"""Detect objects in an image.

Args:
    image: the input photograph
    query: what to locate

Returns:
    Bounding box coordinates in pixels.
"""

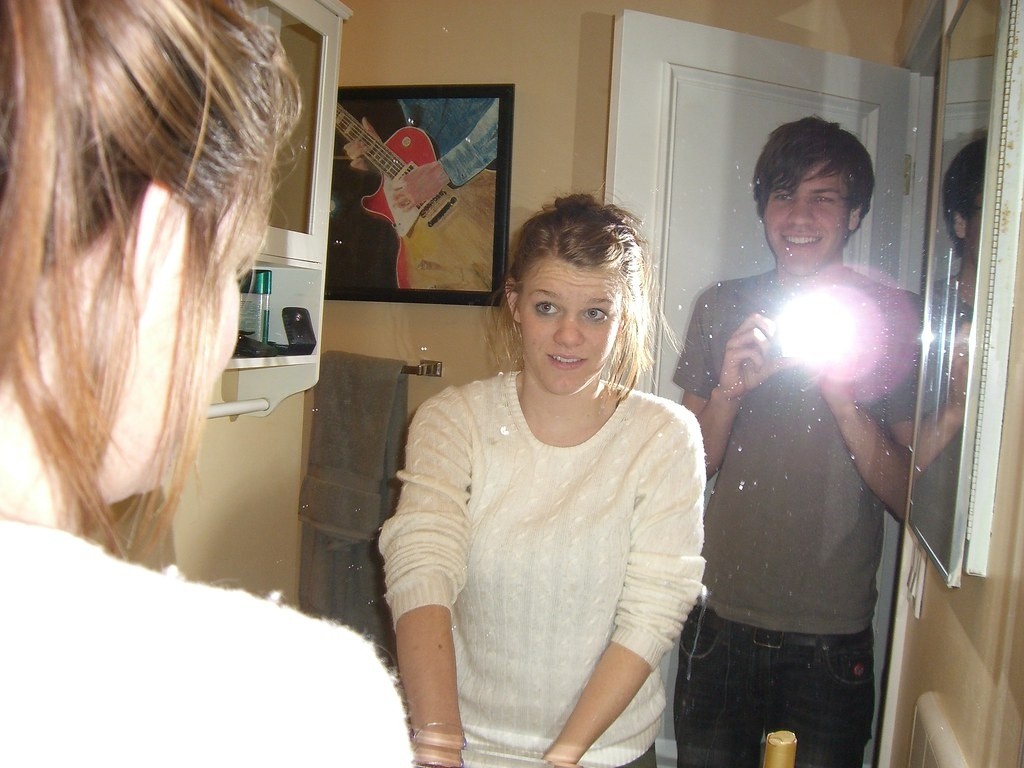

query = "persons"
[909,137,992,571]
[0,0,417,768]
[377,195,706,768]
[675,116,924,768]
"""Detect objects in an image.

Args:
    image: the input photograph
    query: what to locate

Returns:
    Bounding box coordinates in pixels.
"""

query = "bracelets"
[409,723,465,768]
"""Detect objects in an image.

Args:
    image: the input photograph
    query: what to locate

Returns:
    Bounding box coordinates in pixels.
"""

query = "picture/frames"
[322,82,517,306]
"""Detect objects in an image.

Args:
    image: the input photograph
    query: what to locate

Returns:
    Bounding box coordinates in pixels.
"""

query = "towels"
[297,350,410,662]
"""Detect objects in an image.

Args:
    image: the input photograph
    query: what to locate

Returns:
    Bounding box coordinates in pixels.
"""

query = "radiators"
[908,690,969,768]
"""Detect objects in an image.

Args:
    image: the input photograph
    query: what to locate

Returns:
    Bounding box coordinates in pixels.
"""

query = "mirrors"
[905,0,1024,593]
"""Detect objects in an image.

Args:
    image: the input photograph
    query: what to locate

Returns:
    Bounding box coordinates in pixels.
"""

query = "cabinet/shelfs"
[206,1,356,423]
